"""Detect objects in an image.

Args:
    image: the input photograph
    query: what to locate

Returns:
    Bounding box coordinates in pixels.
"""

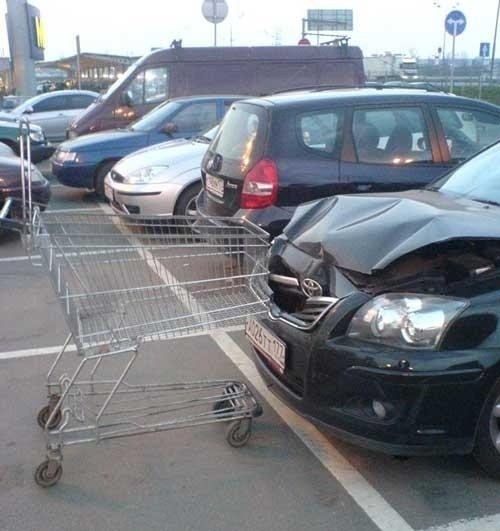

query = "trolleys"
[0,118,275,489]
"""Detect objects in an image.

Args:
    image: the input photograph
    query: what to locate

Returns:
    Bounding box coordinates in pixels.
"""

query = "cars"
[245,139,500,481]
[1,89,254,235]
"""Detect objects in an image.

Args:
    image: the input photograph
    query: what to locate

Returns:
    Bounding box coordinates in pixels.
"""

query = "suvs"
[194,82,500,261]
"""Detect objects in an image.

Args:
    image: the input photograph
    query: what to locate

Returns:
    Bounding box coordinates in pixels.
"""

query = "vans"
[67,37,366,139]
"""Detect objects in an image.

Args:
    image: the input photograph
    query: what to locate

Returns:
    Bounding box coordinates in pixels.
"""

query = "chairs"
[352,121,414,163]
